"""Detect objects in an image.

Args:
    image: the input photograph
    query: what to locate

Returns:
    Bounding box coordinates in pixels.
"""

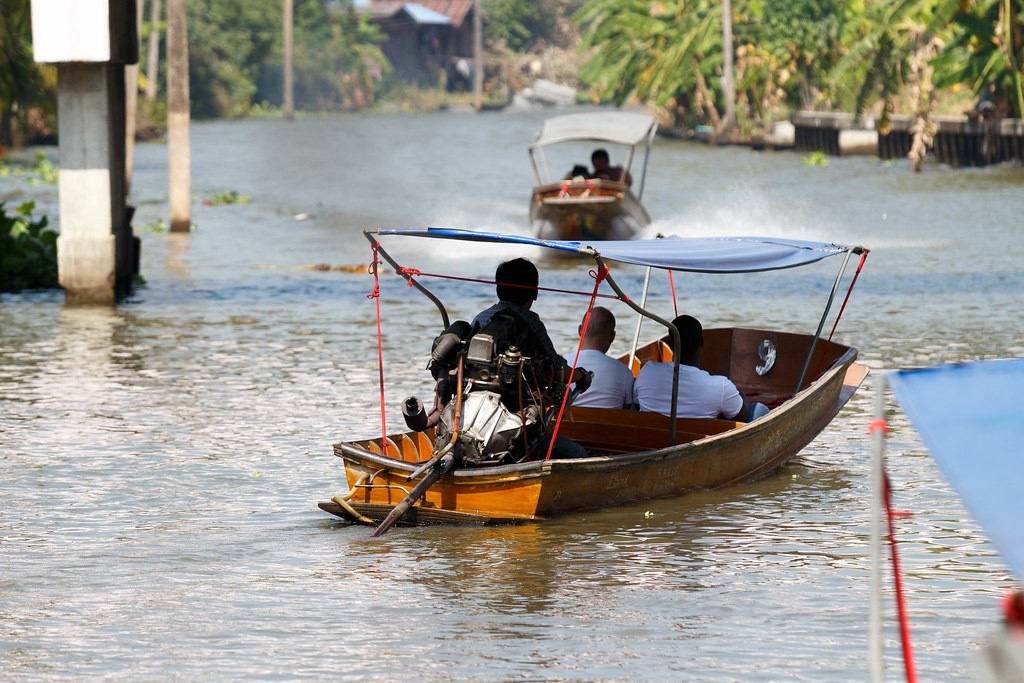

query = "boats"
[313,223,882,541]
[524,108,660,260]
[869,354,1024,683]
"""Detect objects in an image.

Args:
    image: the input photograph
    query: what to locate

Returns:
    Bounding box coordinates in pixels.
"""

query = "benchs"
[553,403,750,455]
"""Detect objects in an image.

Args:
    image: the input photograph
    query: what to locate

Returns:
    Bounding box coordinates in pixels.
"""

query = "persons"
[634,315,769,425]
[472,256,592,459]
[560,305,634,411]
[562,149,632,190]
